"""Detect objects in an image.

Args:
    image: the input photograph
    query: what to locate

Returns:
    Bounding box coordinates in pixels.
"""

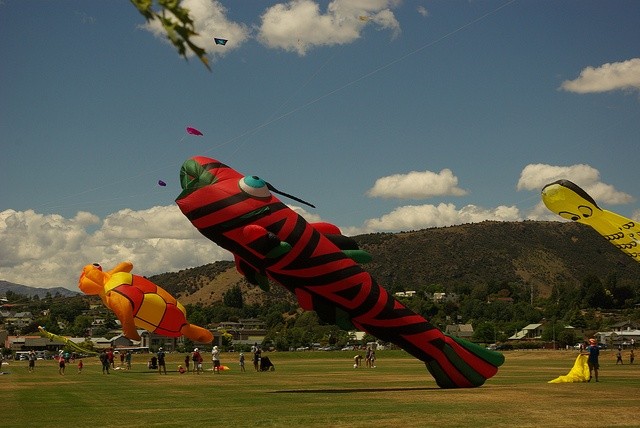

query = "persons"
[185,356,190,373]
[629,349,634,364]
[28,349,36,373]
[177,365,184,373]
[58,354,65,375]
[616,348,623,365]
[77,359,83,374]
[364,346,371,368]
[580,340,589,352]
[108,348,117,368]
[71,354,75,364]
[120,352,125,366]
[106,350,110,363]
[65,351,70,363]
[100,349,110,375]
[59,349,65,360]
[354,354,363,367]
[211,346,220,375]
[239,353,245,372]
[156,346,167,375]
[197,356,204,374]
[191,348,201,375]
[368,351,377,369]
[580,338,599,383]
[253,342,261,372]
[0,348,4,369]
[125,351,131,370]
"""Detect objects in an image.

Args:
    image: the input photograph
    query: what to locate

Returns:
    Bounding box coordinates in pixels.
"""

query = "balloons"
[37,326,99,355]
[78,261,214,345]
[174,156,505,389]
[541,179,640,262]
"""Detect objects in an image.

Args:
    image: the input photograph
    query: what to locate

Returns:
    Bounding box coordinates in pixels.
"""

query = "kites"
[359,15,371,22]
[187,127,203,136]
[159,180,166,186]
[130,0,212,72]
[214,38,228,46]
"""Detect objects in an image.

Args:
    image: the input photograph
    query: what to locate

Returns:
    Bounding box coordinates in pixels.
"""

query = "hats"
[194,348,198,351]
[213,346,218,350]
[589,339,596,344]
